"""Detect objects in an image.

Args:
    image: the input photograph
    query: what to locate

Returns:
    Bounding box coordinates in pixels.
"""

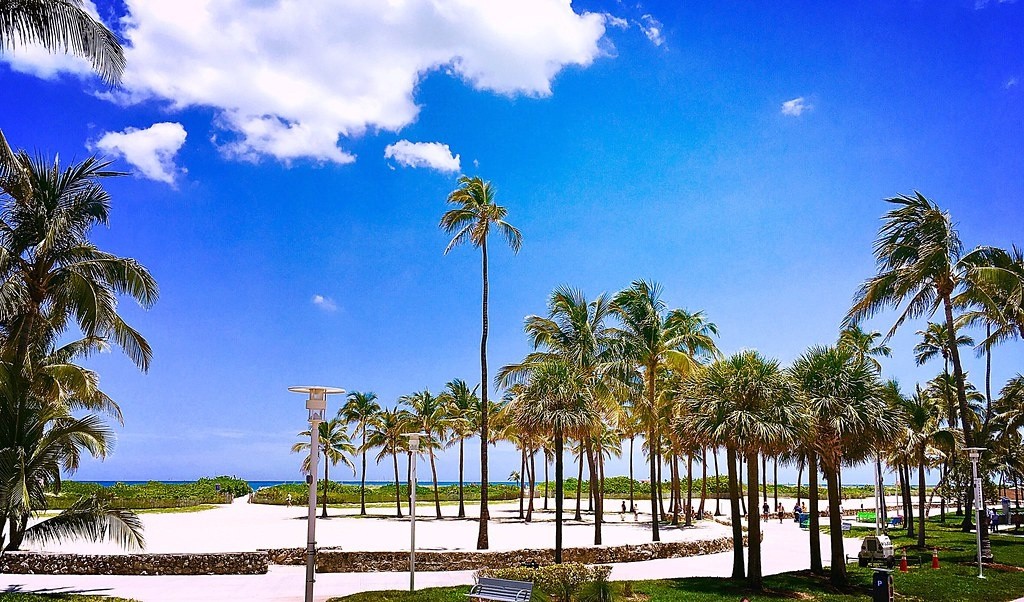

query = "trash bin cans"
[872,567,896,602]
[800,514,808,528]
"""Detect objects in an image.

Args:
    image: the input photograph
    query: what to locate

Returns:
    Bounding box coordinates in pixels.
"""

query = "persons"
[986,508,999,534]
[621,501,695,521]
[287,493,293,507]
[248,487,253,504]
[763,502,807,524]
[231,492,235,504]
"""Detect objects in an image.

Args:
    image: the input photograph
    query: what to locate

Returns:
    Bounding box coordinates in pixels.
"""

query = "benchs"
[463,577,533,602]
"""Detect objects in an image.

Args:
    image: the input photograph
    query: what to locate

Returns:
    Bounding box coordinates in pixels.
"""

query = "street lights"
[287,385,346,602]
[399,433,429,590]
[963,447,987,580]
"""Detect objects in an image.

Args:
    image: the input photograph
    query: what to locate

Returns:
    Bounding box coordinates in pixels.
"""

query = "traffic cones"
[930,546,941,569]
[899,547,909,573]
